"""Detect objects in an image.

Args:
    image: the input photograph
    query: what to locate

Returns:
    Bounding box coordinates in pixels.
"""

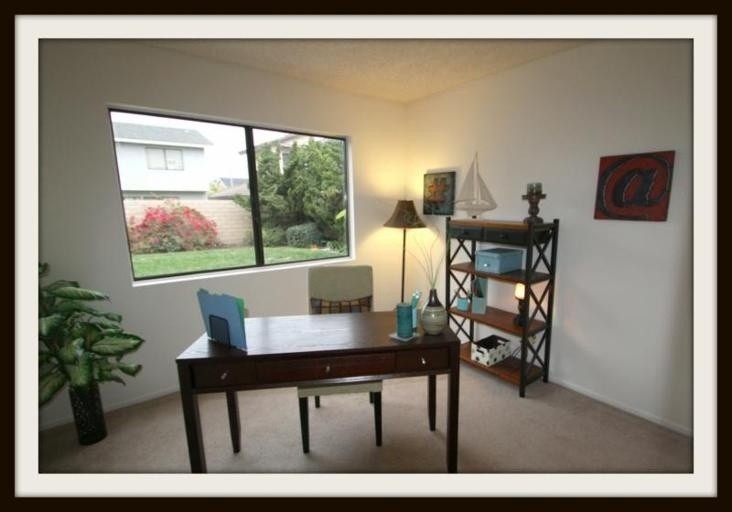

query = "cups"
[412,305,417,332]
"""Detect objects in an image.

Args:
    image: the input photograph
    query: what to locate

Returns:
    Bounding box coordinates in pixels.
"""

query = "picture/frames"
[423,172,455,215]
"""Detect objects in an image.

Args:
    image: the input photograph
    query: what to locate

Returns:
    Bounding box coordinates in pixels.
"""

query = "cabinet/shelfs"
[446,215,559,397]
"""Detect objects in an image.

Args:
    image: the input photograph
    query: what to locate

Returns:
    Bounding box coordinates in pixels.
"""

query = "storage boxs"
[475,248,523,274]
[471,334,512,367]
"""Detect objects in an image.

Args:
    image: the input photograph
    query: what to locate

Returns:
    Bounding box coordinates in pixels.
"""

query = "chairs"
[297,265,382,454]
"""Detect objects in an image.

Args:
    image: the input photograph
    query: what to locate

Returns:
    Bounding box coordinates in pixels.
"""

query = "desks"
[175,309,460,473]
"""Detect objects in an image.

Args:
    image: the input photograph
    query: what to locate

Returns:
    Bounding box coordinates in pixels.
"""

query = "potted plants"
[38,262,145,445]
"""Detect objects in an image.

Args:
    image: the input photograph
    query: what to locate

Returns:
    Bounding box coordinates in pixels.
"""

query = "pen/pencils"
[411,291,422,308]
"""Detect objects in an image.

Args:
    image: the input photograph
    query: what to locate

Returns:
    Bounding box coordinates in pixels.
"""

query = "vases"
[421,289,447,336]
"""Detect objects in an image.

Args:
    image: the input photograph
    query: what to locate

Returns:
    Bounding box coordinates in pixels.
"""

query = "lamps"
[514,282,530,327]
[384,200,427,303]
